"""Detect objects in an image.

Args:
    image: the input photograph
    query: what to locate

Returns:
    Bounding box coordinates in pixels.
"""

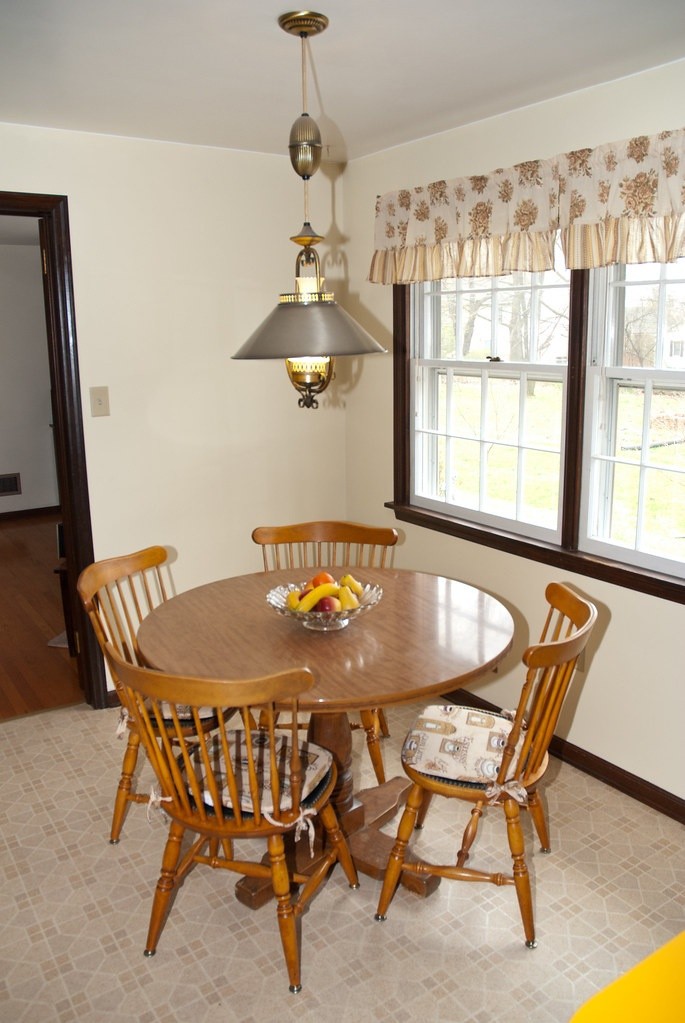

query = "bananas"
[286,583,341,613]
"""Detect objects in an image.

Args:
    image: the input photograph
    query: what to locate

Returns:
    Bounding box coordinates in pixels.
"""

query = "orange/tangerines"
[312,572,335,587]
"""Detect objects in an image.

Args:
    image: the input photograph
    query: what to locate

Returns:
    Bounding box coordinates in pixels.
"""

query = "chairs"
[104,642,363,993]
[77,544,239,844]
[252,521,402,784]
[374,582,599,948]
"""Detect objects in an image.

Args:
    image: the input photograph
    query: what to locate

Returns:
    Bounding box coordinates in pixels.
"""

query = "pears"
[338,574,363,611]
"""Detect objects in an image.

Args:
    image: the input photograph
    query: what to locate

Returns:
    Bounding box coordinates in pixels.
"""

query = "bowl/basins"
[265,582,385,631]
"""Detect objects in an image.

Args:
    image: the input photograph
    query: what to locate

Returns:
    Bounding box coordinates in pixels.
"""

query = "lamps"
[234,12,386,412]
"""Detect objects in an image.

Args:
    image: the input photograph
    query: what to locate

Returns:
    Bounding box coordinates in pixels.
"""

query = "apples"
[312,596,341,613]
[299,589,313,601]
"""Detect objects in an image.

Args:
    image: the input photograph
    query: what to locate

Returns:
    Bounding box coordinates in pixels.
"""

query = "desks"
[137,567,529,912]
